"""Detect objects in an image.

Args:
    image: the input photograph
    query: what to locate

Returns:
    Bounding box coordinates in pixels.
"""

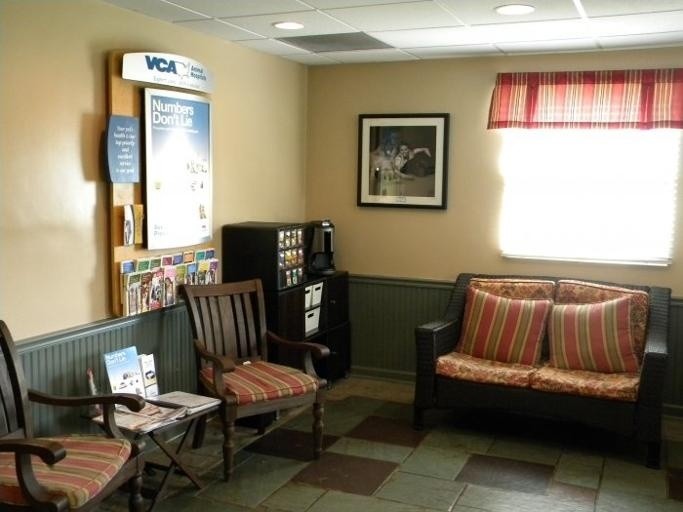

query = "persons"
[393,143,431,180]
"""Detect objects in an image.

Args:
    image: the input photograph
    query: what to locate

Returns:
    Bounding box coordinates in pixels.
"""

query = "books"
[91,345,222,435]
[119,247,218,315]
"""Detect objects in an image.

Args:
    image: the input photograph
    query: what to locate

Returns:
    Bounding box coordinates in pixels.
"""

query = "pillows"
[450,282,555,369]
[538,290,644,378]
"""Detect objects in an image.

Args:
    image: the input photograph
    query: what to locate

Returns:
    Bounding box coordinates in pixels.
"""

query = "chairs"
[0,318,153,512]
[177,275,331,484]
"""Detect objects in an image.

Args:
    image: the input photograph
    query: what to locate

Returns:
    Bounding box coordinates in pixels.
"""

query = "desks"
[75,385,223,512]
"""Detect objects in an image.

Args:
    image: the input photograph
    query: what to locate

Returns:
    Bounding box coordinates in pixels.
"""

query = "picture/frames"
[354,108,453,212]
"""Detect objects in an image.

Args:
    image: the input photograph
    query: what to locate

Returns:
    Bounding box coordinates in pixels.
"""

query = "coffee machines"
[307,219,335,276]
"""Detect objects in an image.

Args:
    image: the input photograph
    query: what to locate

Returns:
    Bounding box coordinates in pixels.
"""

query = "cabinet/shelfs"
[216,266,353,400]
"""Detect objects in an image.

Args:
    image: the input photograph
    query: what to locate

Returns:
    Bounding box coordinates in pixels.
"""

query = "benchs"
[407,267,675,470]
